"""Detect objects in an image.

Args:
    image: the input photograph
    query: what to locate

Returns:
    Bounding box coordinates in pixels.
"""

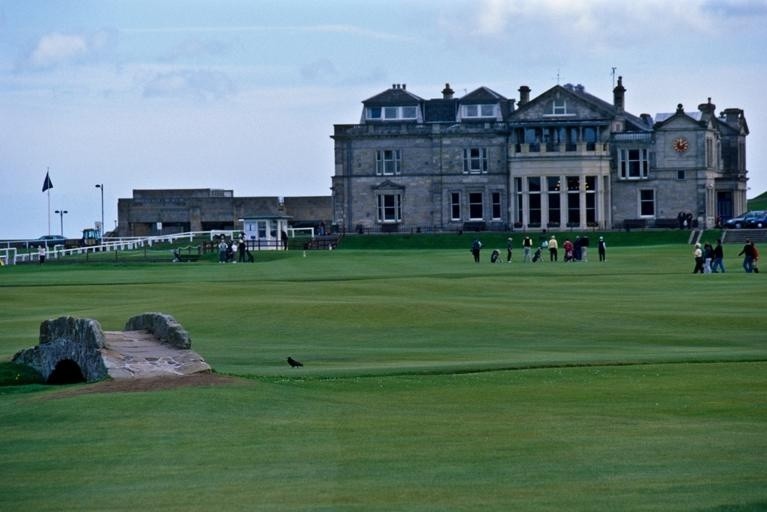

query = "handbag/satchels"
[752,260,758,269]
[491,249,500,263]
[532,247,542,263]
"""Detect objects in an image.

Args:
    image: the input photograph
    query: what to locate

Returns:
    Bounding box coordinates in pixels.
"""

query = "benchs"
[623,219,646,232]
[380,223,399,232]
[655,218,680,231]
[463,222,485,231]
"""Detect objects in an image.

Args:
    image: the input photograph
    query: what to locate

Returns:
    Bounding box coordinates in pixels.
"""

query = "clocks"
[672,136,689,153]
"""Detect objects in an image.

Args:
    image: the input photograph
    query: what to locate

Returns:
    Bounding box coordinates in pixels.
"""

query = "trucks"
[64,228,101,254]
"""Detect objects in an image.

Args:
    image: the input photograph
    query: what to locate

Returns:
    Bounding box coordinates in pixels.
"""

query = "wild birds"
[285,356,303,368]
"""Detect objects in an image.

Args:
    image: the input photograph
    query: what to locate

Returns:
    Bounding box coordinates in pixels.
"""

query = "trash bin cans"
[356,225,362,233]
[330,223,339,232]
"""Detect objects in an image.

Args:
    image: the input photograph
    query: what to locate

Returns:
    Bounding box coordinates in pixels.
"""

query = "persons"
[490,249,502,264]
[539,228,549,249]
[597,236,607,263]
[522,235,532,263]
[748,240,758,275]
[685,210,693,230]
[736,237,754,272]
[547,234,558,262]
[216,237,245,264]
[36,243,46,268]
[282,232,288,252]
[506,236,514,264]
[580,234,589,262]
[572,235,581,262]
[676,209,686,230]
[531,246,543,263]
[561,238,572,262]
[470,238,481,264]
[690,238,725,274]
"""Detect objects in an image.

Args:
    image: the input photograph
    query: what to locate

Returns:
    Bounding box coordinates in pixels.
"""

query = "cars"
[22,235,67,249]
[724,210,767,229]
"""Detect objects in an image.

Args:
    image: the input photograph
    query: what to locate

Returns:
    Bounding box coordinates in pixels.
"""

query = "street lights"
[94,183,104,247]
[54,210,68,235]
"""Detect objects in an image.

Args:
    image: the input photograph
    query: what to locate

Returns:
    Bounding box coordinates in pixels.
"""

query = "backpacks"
[564,240,572,251]
